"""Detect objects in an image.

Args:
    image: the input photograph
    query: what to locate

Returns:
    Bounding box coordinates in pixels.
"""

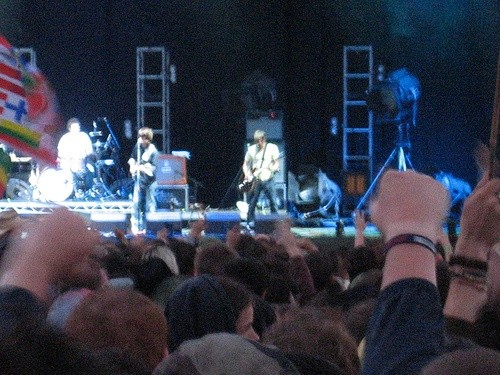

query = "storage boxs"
[150,185,189,212]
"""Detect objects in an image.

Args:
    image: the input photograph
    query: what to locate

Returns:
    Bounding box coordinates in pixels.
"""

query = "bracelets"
[447,253,490,292]
[383,235,438,257]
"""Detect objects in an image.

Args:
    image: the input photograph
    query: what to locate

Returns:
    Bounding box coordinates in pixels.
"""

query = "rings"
[201,226,205,231]
[356,215,359,217]
[86,225,102,238]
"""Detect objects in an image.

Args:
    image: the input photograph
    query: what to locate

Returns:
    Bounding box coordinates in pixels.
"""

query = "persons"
[55,117,102,199]
[0,141,500,375]
[127,125,157,237]
[241,129,281,231]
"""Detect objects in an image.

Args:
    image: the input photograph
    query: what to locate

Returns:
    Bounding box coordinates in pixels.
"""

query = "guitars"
[238,151,286,192]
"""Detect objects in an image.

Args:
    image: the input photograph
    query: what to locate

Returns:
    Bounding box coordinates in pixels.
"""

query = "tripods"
[78,135,116,201]
[352,123,415,219]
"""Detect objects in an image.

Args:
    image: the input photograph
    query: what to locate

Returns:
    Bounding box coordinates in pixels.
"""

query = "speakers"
[145,212,182,236]
[204,212,241,234]
[91,211,131,234]
[255,215,291,235]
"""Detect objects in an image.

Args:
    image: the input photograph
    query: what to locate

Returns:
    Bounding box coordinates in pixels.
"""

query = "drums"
[37,169,75,201]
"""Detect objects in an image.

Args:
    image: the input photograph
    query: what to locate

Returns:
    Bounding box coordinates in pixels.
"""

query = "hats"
[0,207,31,236]
[152,332,300,375]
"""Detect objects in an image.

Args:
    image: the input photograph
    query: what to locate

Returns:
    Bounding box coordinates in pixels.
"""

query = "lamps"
[354,67,422,210]
